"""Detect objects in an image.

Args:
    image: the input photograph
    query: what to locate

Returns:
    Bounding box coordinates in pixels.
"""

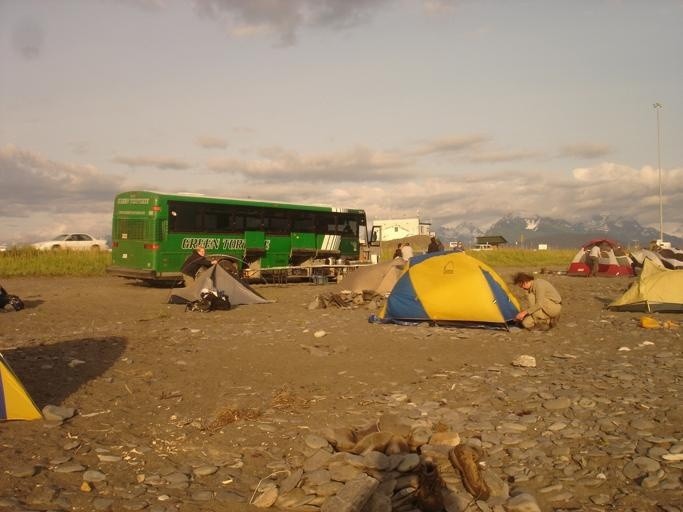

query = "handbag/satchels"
[187,291,231,312]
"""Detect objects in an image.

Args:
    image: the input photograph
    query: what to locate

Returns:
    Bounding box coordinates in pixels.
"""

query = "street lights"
[653,103,663,239]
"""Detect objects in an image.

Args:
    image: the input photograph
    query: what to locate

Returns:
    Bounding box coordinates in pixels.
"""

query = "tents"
[0,353,45,423]
[605,247,683,314]
[375,249,521,331]
[565,237,636,278]
[169,254,279,305]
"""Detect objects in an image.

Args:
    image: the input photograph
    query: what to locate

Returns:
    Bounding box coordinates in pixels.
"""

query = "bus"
[111,192,381,285]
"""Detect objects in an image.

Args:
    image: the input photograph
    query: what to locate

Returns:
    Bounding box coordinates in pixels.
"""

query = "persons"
[428,237,438,252]
[513,272,562,330]
[589,243,602,278]
[393,243,402,259]
[179,246,218,286]
[453,242,465,252]
[402,242,413,260]
[437,240,444,250]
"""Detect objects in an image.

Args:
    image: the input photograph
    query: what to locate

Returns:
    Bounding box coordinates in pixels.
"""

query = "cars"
[33,233,110,251]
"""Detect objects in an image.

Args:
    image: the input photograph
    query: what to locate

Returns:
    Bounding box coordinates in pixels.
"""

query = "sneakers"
[550,314,559,328]
[529,323,549,331]
